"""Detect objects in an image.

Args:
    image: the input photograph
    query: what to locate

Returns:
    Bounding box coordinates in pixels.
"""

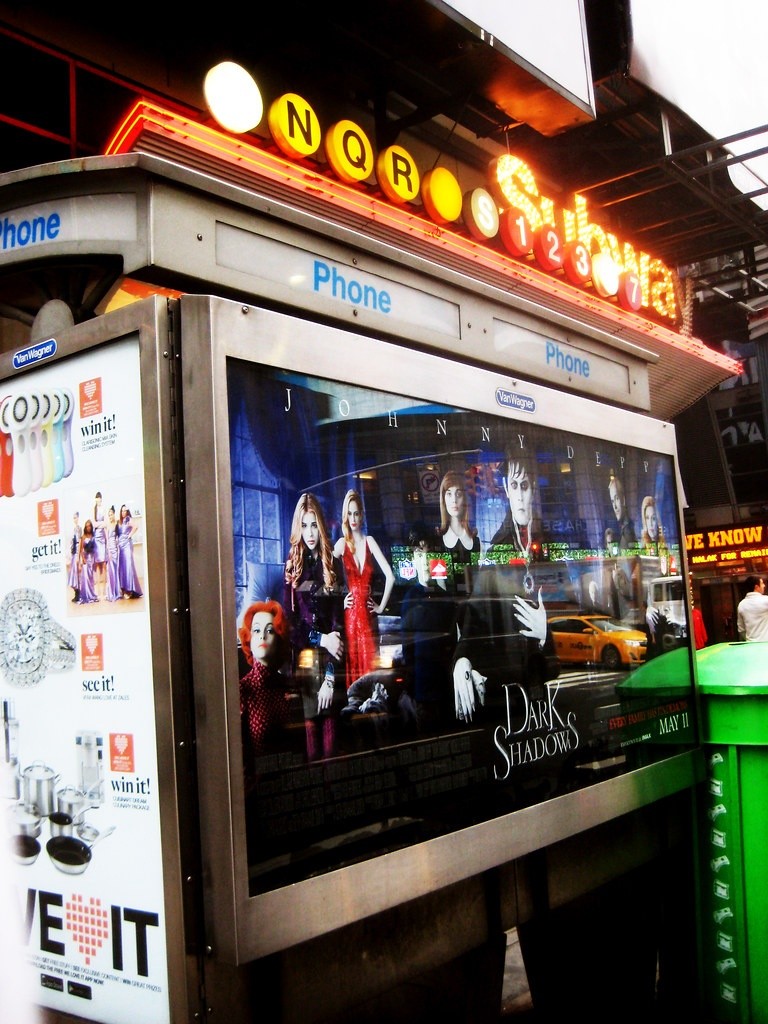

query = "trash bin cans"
[615,646,691,768]
[697,634,768,1023]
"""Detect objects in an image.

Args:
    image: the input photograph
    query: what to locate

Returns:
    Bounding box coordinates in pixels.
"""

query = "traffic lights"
[533,544,543,561]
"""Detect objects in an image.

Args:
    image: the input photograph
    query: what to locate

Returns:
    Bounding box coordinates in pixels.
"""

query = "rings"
[372,603,374,606]
[464,671,472,680]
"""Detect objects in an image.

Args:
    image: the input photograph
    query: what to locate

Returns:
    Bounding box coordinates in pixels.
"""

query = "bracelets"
[324,676,335,688]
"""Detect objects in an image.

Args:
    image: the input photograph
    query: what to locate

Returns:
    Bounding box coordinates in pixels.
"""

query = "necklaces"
[512,518,535,594]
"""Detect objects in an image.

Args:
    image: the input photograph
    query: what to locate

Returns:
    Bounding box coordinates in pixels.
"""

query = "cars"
[649,576,685,625]
[548,615,648,666]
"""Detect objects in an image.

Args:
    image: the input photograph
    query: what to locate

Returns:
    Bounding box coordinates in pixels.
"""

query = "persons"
[66,491,142,604]
[236,442,677,766]
[736,576,768,642]
